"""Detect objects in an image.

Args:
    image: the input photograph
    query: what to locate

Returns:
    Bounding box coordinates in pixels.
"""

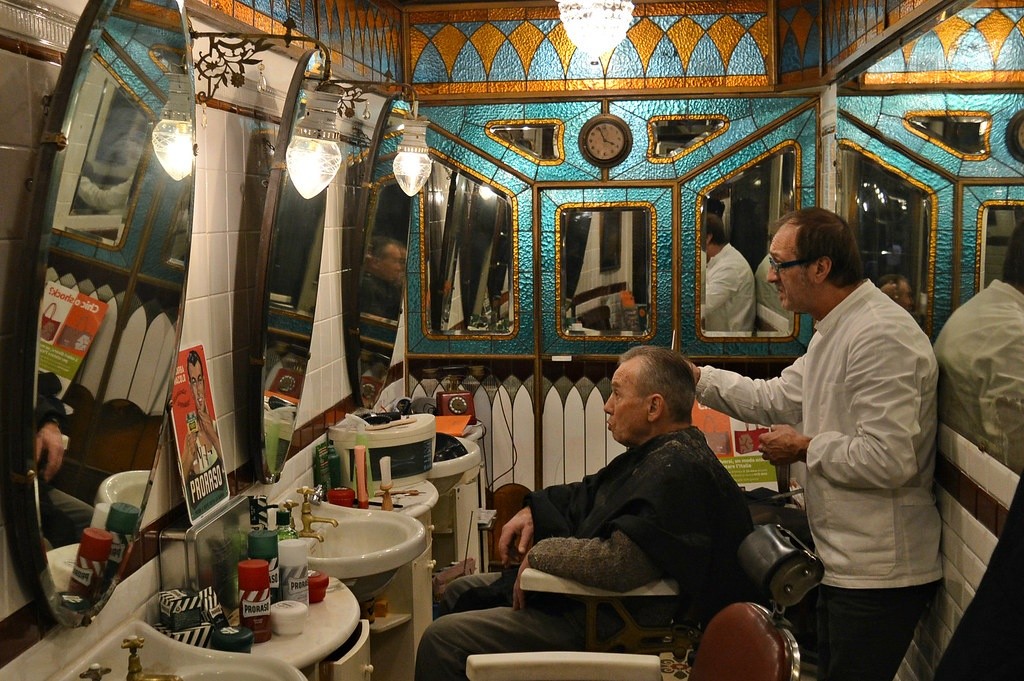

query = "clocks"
[1004,108,1024,164]
[579,113,633,170]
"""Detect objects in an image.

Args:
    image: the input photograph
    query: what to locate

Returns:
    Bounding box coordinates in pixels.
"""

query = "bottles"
[67,502,142,614]
[275,510,299,541]
[276,539,312,608]
[249,530,281,610]
[237,558,273,644]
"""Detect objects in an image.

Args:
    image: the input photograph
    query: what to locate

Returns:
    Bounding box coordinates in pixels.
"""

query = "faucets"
[79,663,112,681]
[122,636,182,681]
[297,485,338,542]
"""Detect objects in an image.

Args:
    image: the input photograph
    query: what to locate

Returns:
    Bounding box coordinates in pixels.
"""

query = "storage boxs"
[154,588,230,652]
[37,279,109,400]
[714,430,779,496]
[314,439,341,499]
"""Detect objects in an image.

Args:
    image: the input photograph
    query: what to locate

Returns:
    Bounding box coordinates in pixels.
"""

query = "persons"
[700,214,756,333]
[414,347,754,681]
[77,173,134,212]
[359,236,406,318]
[34,389,95,546]
[933,217,1024,476]
[875,275,911,312]
[755,252,789,317]
[682,207,944,681]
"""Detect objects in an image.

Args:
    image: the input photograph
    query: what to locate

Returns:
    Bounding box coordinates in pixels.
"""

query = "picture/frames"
[599,210,621,272]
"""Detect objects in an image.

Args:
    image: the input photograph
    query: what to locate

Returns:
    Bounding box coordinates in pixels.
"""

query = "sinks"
[307,507,427,579]
[433,437,481,476]
[183,642,309,681]
[95,469,150,506]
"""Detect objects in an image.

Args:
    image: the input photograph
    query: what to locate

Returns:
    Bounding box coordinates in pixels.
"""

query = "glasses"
[769,253,835,275]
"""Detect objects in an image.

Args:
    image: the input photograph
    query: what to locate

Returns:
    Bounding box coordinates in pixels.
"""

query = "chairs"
[467,495,827,681]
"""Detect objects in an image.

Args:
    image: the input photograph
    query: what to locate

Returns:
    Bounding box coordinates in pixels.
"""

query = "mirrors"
[836,137,938,342]
[51,50,159,250]
[5,0,657,627]
[484,119,565,166]
[160,183,194,274]
[647,0,1024,476]
[973,200,1024,294]
[356,172,410,329]
[904,110,992,161]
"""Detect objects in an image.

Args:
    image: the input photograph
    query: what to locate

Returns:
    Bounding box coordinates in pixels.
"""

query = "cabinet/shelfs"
[300,469,482,681]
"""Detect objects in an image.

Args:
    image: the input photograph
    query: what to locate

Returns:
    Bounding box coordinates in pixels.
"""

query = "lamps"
[190,19,344,201]
[330,79,432,197]
[149,41,194,181]
[555,0,636,65]
